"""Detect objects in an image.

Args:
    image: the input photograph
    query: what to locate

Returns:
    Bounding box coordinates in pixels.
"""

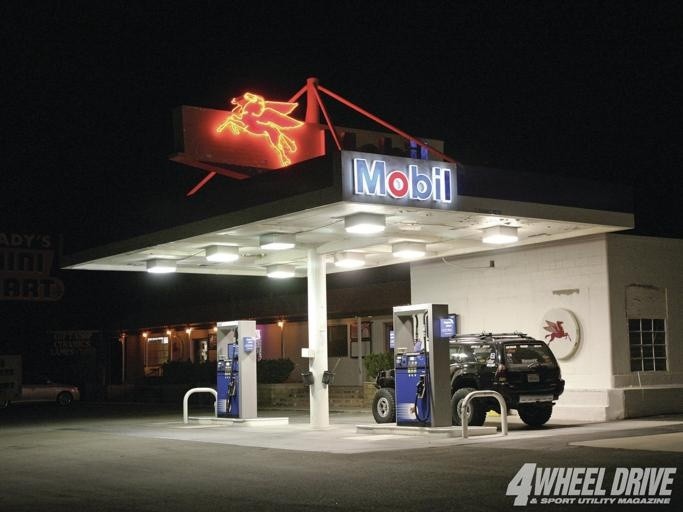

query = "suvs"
[372,333,565,426]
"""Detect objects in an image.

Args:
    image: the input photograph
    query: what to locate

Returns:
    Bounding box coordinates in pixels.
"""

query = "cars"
[0,375,81,409]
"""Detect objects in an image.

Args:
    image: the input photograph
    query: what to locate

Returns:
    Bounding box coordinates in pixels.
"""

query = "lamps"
[481,225,518,245]
[146,212,427,279]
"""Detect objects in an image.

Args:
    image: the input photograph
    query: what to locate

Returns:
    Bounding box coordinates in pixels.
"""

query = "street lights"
[119,332,126,384]
